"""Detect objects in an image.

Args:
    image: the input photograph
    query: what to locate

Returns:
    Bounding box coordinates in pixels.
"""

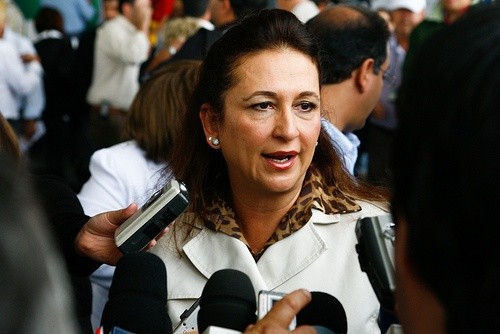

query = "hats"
[392,0,427,14]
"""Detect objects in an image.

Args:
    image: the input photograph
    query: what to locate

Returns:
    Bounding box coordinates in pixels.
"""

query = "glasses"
[373,63,398,87]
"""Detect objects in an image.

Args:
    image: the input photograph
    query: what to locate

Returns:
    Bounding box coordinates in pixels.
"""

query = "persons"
[27,8,75,133]
[370,0,425,149]
[98,10,410,334]
[88,0,151,147]
[0,1,500,333]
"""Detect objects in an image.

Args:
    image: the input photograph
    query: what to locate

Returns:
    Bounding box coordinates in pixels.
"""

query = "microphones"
[100,252,258,334]
[295,291,347,334]
